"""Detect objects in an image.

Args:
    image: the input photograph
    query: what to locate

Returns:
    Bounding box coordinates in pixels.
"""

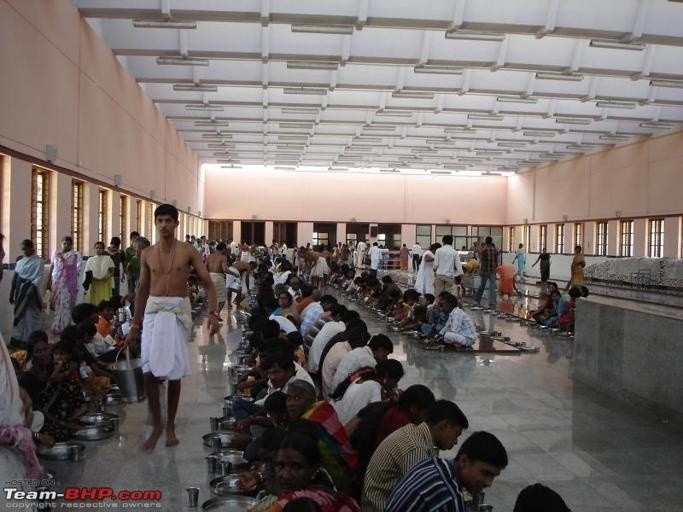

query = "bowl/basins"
[41,386,121,461]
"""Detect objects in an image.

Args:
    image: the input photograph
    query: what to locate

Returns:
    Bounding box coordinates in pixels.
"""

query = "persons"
[0,204,589,512]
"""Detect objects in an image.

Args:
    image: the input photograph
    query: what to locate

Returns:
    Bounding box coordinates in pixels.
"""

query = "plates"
[474,323,538,353]
[466,303,574,339]
[353,298,439,350]
[199,343,253,511]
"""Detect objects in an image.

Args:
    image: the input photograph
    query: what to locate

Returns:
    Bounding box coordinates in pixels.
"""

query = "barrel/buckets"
[478,330,495,352]
[106,343,146,404]
[229,276,242,293]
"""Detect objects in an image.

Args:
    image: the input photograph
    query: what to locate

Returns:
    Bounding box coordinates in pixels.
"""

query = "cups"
[184,484,199,508]
